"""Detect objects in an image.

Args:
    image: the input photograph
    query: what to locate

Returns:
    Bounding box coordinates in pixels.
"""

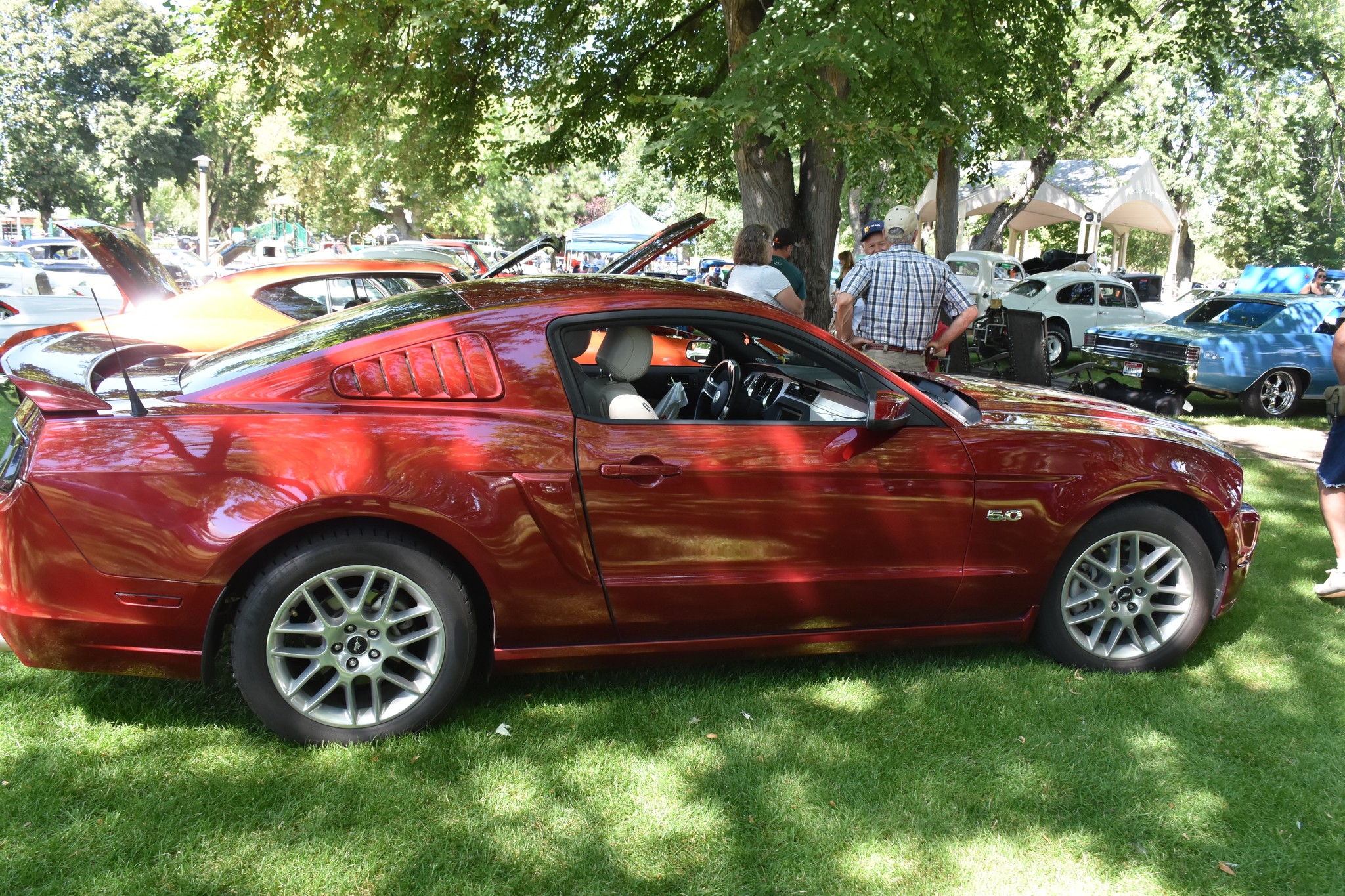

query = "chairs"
[1007,308,1098,395]
[582,323,659,420]
[561,327,592,388]
[940,308,1009,378]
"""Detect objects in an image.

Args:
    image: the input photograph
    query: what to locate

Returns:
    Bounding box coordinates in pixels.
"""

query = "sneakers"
[1313,569,1345,598]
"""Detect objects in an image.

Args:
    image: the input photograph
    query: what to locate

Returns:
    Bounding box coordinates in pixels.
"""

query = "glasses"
[1315,275,1327,278]
[768,241,775,247]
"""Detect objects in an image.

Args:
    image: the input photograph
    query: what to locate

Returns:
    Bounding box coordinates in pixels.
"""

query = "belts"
[865,344,926,356]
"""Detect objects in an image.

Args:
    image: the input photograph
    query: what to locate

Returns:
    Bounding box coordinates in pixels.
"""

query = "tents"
[563,201,697,281]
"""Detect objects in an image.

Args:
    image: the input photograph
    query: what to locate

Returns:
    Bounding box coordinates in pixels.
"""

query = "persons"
[60,251,71,260]
[927,320,949,372]
[201,253,227,281]
[188,241,197,253]
[829,250,865,337]
[860,221,889,304]
[1071,267,1102,302]
[725,223,803,315]
[1314,312,1345,598]
[769,228,808,319]
[835,206,979,372]
[1110,267,1125,294]
[1300,268,1327,295]
[556,252,690,281]
[705,267,722,286]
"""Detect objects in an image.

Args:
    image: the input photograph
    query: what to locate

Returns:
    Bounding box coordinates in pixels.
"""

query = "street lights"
[192,154,213,266]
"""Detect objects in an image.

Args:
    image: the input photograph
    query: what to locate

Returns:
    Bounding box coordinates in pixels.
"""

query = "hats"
[714,267,721,275]
[884,205,920,239]
[861,220,884,242]
[773,228,801,247]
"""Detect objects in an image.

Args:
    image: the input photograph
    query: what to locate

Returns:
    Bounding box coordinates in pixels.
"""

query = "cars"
[2,273,1259,748]
[972,270,1148,371]
[943,251,1032,330]
[1114,272,1240,323]
[1078,265,1345,415]
[0,212,806,420]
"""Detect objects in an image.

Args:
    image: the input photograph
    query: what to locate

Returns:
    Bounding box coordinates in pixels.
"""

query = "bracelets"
[841,330,854,341]
